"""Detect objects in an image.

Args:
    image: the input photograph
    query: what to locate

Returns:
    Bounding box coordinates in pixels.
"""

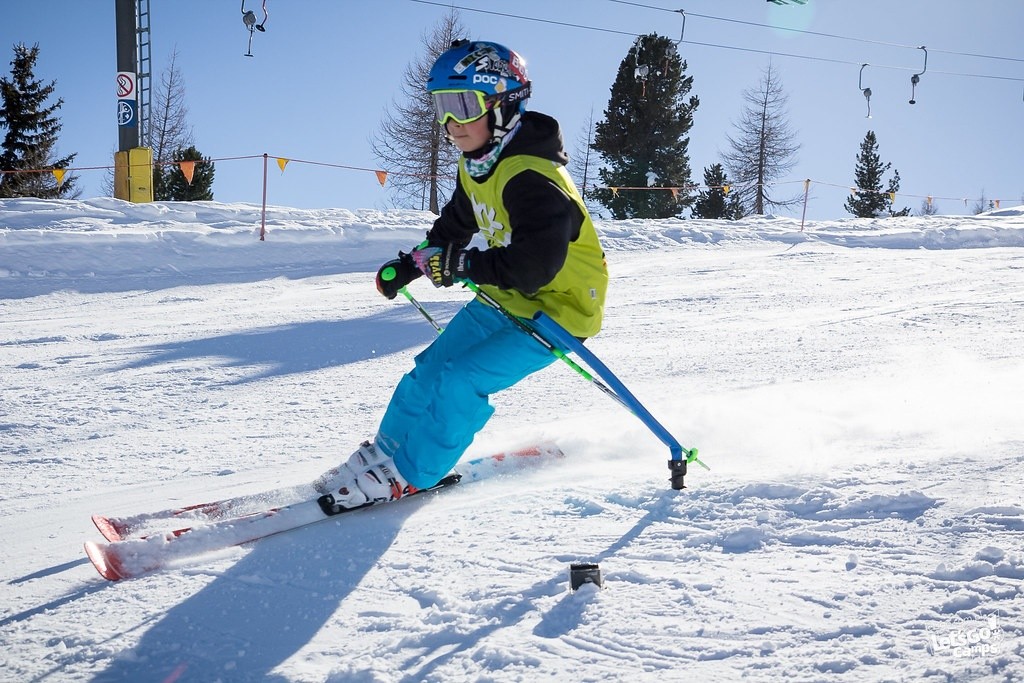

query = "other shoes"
[348,440,390,476]
[356,457,409,500]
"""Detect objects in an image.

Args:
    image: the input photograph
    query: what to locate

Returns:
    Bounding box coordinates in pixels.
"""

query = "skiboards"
[81,438,564,582]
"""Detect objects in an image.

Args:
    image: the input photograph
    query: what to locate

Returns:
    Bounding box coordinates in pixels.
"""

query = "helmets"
[426,39,534,147]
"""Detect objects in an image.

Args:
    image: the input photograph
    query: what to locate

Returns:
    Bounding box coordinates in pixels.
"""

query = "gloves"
[375,250,416,300]
[413,243,469,288]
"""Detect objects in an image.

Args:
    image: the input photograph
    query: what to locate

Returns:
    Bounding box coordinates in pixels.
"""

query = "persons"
[313,35,608,506]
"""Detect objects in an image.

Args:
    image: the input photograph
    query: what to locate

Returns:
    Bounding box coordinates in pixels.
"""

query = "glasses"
[431,89,494,126]
